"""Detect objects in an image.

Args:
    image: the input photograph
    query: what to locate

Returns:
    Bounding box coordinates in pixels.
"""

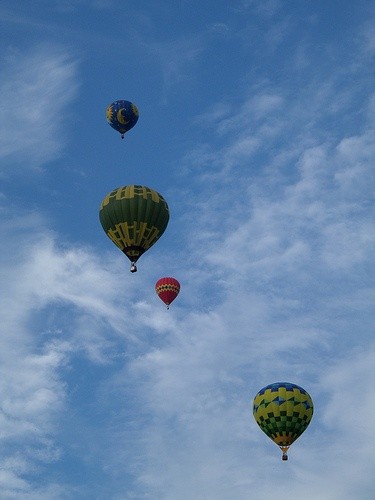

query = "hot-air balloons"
[252,382,315,461]
[106,100,140,139]
[97,184,169,273]
[154,277,181,310]
